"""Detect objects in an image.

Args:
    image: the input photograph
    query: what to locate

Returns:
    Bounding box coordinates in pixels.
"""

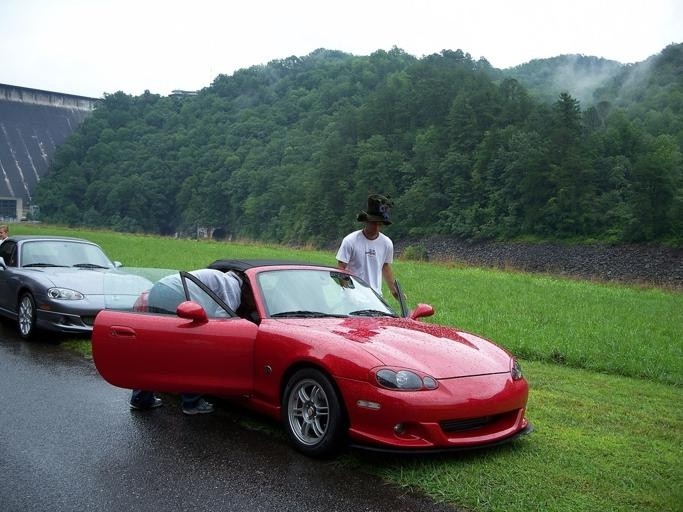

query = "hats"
[355,190,394,226]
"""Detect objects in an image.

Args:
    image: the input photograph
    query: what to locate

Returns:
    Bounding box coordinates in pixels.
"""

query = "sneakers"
[182,399,216,415]
[129,395,163,410]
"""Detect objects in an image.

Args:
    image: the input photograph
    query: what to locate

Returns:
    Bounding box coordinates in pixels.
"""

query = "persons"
[0,225,9,244]
[336,220,406,304]
[128,267,242,414]
